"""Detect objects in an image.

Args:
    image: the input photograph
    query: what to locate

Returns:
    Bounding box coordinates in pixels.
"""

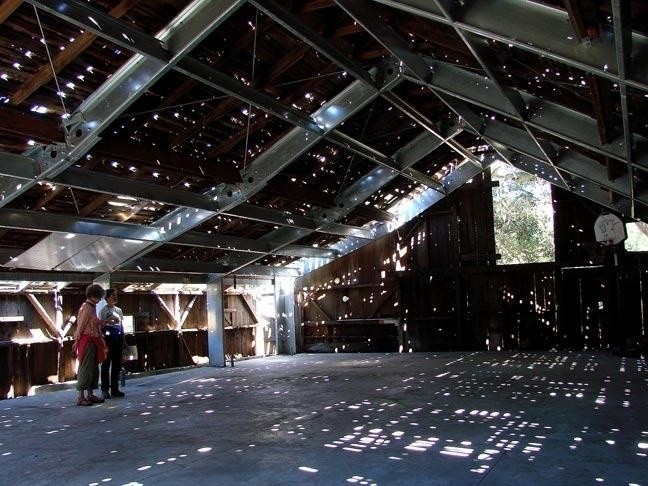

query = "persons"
[97,288,128,398]
[71,284,105,406]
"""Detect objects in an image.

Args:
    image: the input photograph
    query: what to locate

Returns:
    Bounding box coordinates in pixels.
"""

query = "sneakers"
[77,390,125,406]
